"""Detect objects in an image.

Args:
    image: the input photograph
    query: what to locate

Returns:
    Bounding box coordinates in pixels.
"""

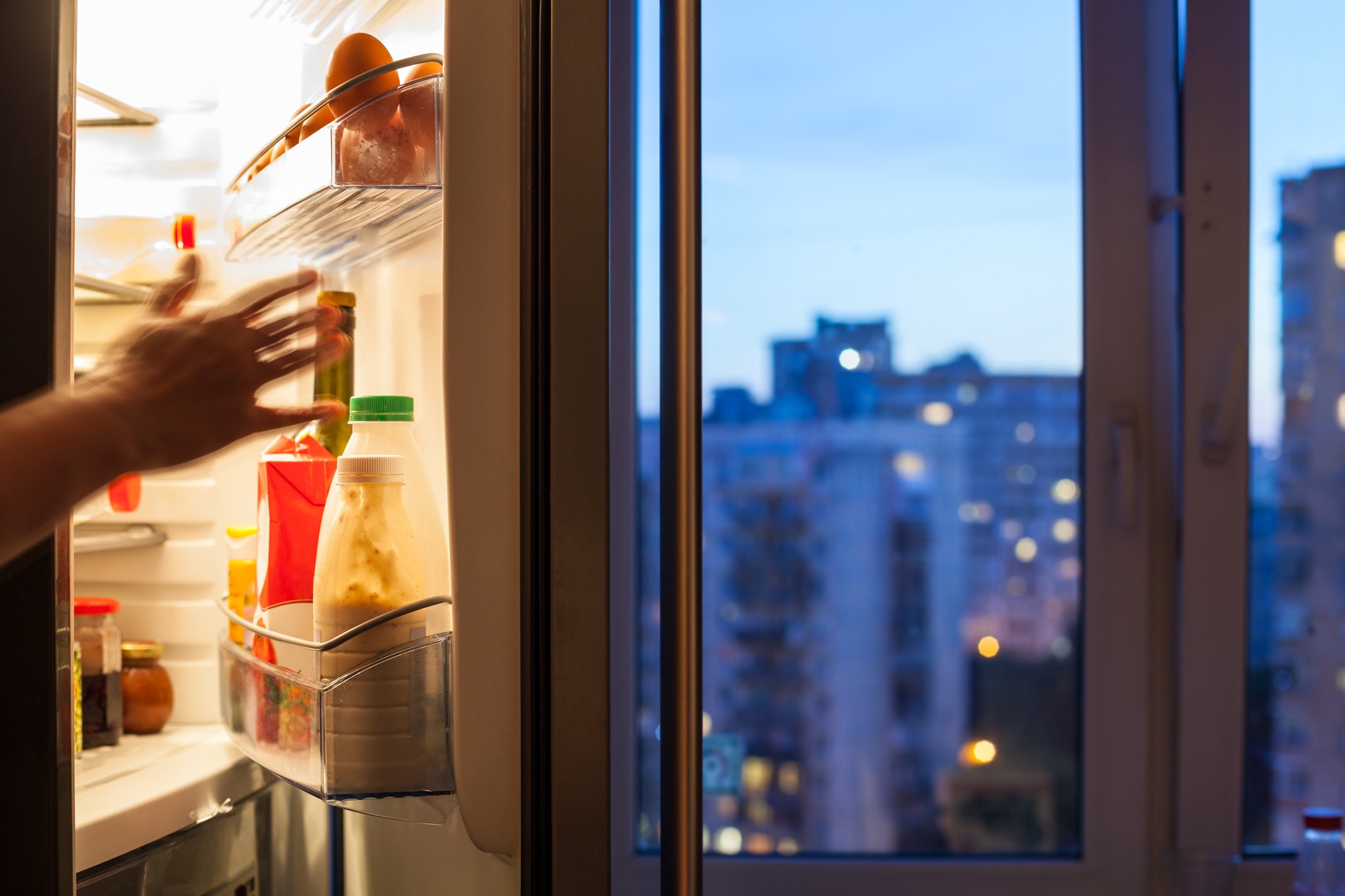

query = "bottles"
[313,292,354,457]
[315,455,430,800]
[317,395,453,697]
[73,596,124,749]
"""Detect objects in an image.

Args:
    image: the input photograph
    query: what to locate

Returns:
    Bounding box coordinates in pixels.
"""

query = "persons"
[0,253,352,562]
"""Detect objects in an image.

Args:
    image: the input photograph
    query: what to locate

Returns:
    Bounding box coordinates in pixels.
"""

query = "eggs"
[249,33,443,184]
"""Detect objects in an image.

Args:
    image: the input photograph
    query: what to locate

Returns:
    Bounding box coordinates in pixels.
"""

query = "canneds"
[121,639,174,736]
